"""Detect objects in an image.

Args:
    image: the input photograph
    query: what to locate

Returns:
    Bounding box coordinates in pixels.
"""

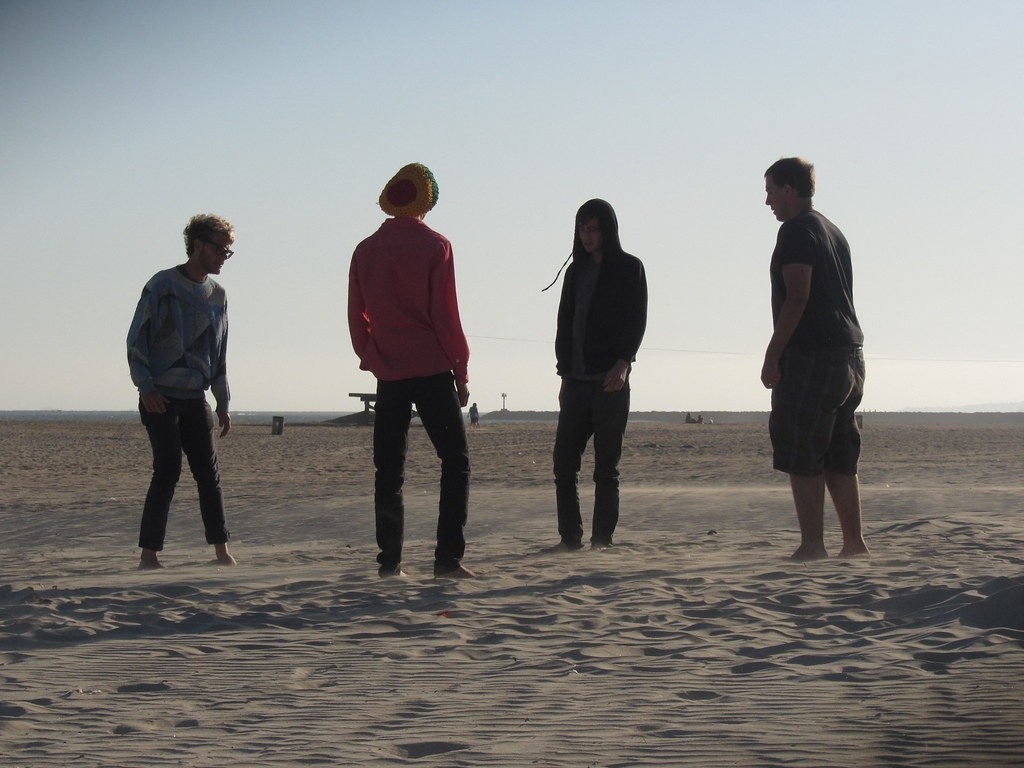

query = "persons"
[761,158,866,558]
[348,163,476,580]
[467,401,480,428]
[543,198,647,550]
[126,215,237,567]
[685,412,703,423]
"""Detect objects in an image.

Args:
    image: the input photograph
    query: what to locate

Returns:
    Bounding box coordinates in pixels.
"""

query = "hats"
[379,162,439,216]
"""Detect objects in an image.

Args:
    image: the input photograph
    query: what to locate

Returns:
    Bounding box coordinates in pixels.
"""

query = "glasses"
[208,240,234,259]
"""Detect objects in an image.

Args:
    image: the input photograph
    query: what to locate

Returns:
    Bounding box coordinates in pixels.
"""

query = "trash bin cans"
[272,416,284,435]
